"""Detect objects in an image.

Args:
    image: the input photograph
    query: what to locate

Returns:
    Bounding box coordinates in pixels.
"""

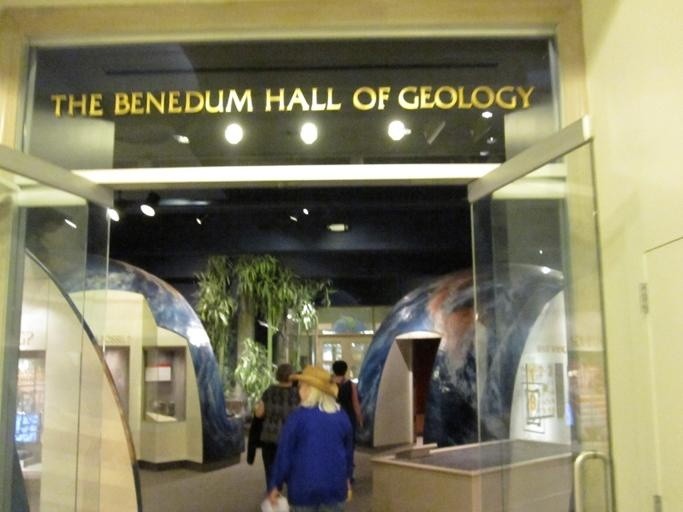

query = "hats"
[289,365,340,397]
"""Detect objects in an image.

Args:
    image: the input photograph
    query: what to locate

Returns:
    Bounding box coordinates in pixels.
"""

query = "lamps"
[109,190,160,224]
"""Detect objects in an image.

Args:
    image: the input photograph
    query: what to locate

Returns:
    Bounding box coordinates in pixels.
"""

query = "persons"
[254,364,302,494]
[329,362,361,485]
[265,361,355,511]
